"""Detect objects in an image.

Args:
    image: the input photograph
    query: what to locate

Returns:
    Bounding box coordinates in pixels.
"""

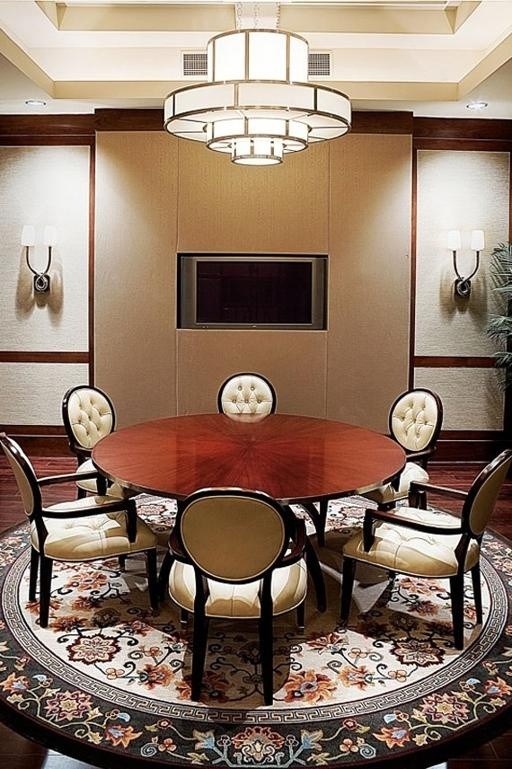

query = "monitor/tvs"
[178,255,327,330]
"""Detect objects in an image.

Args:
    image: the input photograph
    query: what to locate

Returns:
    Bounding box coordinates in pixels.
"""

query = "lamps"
[448,228,486,297]
[19,225,61,293]
[159,4,352,168]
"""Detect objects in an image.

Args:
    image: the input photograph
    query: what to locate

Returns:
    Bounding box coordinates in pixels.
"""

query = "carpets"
[0,493,509,769]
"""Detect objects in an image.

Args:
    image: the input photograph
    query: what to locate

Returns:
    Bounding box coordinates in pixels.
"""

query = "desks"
[88,413,409,635]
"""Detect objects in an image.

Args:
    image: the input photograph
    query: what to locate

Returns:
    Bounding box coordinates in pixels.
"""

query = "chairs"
[337,450,512,648]
[319,387,445,531]
[217,373,278,415]
[1,429,163,628]
[62,385,143,500]
[158,484,310,706]
